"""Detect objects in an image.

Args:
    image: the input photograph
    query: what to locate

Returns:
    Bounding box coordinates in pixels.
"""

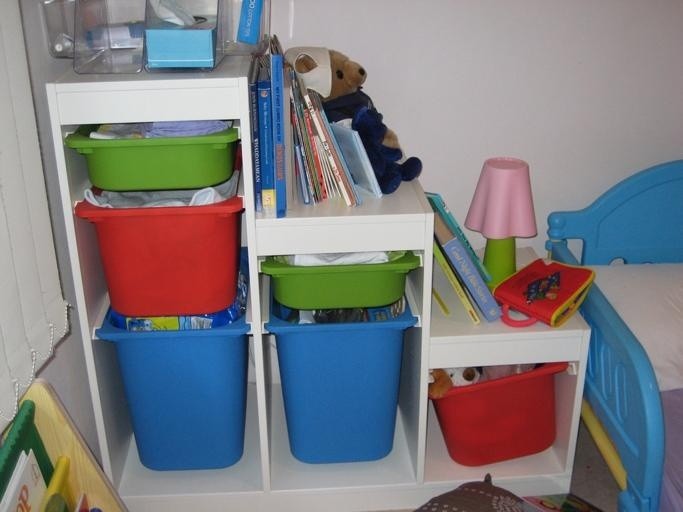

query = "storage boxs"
[65,126,238,193]
[264,282,418,464]
[429,362,569,466]
[76,145,243,317]
[261,255,421,312]
[96,246,250,471]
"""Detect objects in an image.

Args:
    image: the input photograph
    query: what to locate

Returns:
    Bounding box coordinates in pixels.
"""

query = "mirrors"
[73,0,147,74]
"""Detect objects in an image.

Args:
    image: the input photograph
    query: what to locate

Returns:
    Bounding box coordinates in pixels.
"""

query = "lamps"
[464,157,537,284]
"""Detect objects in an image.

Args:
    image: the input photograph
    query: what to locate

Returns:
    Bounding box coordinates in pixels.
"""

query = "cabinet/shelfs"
[45,55,593,510]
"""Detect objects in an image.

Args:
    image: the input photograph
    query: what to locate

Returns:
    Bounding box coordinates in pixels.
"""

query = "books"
[74,490,92,512]
[245,26,380,221]
[434,239,478,324]
[425,192,493,281]
[0,449,47,511]
[432,211,501,322]
[522,489,605,512]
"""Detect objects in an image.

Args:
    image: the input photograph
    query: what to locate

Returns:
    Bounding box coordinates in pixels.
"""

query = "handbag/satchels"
[492,257,594,328]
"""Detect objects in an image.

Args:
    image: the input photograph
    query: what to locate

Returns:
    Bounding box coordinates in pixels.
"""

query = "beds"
[545,159,683,512]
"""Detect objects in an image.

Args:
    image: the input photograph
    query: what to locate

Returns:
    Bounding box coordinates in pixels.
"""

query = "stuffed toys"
[284,40,420,195]
[423,359,482,401]
